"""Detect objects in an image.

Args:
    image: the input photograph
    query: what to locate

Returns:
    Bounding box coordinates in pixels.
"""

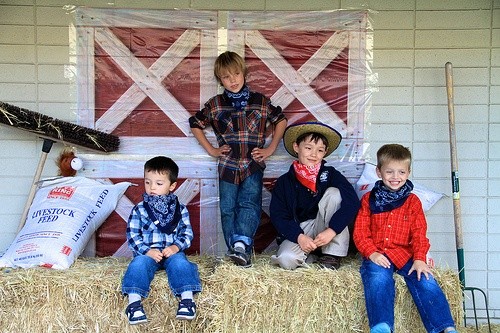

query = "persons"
[353,144,460,333]
[189,51,288,268]
[269,121,360,270]
[121,156,202,325]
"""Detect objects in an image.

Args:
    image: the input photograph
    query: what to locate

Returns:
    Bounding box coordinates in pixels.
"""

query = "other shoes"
[316,252,344,271]
[226,247,252,268]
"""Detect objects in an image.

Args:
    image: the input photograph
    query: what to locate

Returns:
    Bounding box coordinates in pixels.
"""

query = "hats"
[283,122,342,159]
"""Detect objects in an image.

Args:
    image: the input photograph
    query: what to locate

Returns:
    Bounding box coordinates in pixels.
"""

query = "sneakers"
[176,299,197,320]
[125,300,148,325]
